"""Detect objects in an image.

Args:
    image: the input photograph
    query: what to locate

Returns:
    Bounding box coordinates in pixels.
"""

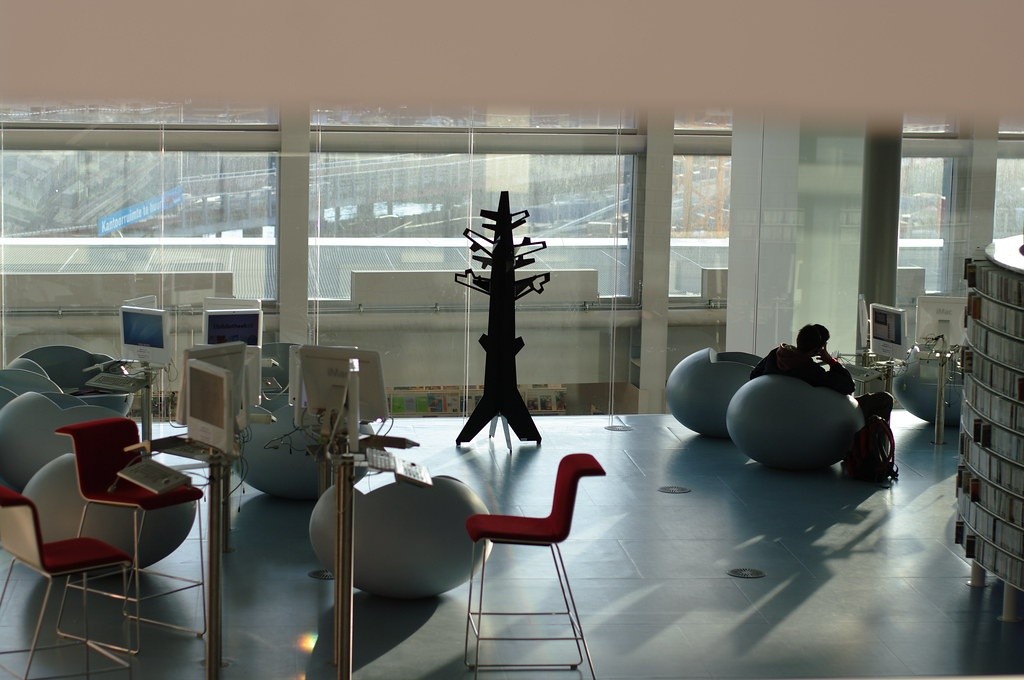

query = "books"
[956,257,1024,593]
[387,392,567,412]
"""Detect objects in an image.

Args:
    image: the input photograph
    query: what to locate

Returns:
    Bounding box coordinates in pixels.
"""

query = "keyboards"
[85,373,147,393]
[262,376,282,392]
[117,460,192,494]
[367,447,394,471]
[396,456,433,486]
[842,364,882,382]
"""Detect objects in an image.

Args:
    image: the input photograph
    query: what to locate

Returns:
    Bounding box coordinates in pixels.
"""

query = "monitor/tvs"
[120,306,171,365]
[870,303,908,360]
[176,297,262,453]
[915,295,967,346]
[300,344,389,422]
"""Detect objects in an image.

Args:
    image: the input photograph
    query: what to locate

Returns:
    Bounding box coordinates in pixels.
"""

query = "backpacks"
[844,414,899,481]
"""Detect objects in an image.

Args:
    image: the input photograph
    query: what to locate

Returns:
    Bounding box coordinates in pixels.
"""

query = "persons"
[591,399,605,415]
[749,324,893,476]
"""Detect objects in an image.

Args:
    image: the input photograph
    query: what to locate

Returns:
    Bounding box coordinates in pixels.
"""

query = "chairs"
[0,484,134,680]
[465,453,607,679]
[0,392,128,485]
[893,372,964,425]
[726,376,867,472]
[237,341,375,493]
[664,345,764,434]
[19,451,198,570]
[309,474,493,599]
[5,345,134,418]
[54,418,207,654]
[0,365,64,405]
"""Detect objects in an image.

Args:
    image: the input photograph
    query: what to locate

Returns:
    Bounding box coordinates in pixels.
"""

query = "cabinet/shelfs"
[948,234,1024,621]
